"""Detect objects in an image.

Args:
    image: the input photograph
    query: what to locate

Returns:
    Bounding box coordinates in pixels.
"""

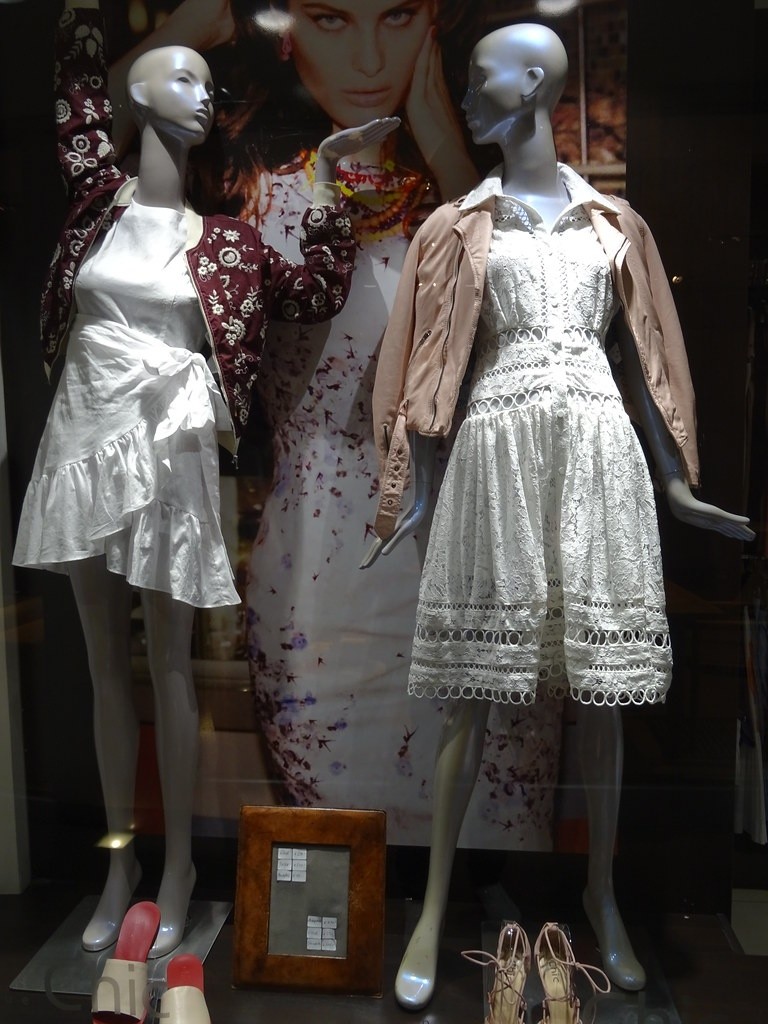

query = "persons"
[132,0,559,863]
[14,0,402,964]
[363,21,753,1007]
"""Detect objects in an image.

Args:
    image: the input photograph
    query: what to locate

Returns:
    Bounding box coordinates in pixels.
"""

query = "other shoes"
[460,921,531,1024]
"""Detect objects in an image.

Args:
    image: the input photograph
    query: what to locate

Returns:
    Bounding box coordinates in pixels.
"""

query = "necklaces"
[301,145,431,245]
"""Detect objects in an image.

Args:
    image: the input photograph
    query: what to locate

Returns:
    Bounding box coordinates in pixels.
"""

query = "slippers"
[160,954,211,1024]
[91,902,160,1024]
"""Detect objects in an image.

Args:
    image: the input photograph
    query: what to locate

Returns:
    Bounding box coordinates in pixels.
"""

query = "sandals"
[534,922,610,1024]
